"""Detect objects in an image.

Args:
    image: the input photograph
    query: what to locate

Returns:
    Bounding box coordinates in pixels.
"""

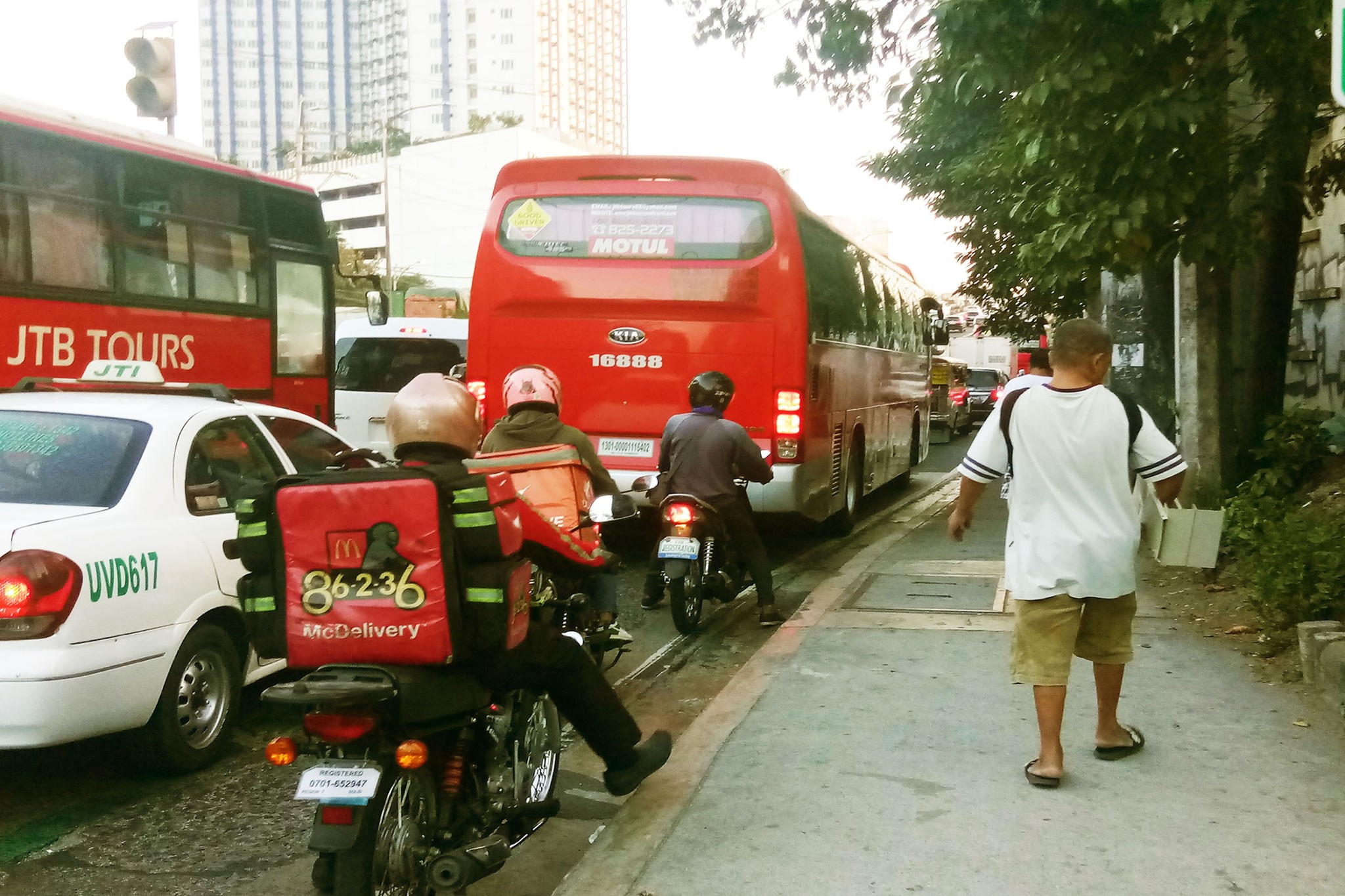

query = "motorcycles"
[657,449,772,634]
[260,474,658,896]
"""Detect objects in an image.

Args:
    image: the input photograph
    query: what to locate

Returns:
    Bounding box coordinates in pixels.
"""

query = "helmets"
[385,372,480,460]
[502,364,562,416]
[688,372,735,412]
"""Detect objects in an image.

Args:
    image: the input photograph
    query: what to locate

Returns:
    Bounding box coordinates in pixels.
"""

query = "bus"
[0,104,388,471]
[466,156,948,539]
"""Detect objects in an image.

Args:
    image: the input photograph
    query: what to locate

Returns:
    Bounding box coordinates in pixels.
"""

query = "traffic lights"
[124,35,178,118]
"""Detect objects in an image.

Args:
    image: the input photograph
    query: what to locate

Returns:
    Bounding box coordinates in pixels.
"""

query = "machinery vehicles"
[927,356,972,444]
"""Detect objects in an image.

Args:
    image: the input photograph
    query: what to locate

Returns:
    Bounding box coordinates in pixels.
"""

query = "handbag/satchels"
[645,471,671,505]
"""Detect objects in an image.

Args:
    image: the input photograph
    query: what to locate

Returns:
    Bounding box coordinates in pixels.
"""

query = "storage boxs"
[261,445,595,674]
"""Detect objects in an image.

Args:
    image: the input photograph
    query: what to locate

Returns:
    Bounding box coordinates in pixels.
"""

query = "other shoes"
[602,730,674,796]
[594,622,634,645]
[759,611,785,626]
[640,594,665,609]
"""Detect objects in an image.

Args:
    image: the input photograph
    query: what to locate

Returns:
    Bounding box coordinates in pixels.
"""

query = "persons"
[641,370,786,625]
[481,364,632,642]
[387,372,673,798]
[993,348,1054,501]
[947,318,1189,783]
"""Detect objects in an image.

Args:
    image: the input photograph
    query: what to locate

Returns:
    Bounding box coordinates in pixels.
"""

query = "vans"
[334,314,471,469]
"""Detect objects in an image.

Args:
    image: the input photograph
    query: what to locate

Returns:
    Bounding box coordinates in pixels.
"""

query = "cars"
[855,371,932,410]
[966,367,1005,420]
[0,389,382,772]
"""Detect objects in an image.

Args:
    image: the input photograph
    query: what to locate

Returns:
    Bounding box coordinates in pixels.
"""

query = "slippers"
[1025,758,1060,786]
[1094,723,1144,760]
